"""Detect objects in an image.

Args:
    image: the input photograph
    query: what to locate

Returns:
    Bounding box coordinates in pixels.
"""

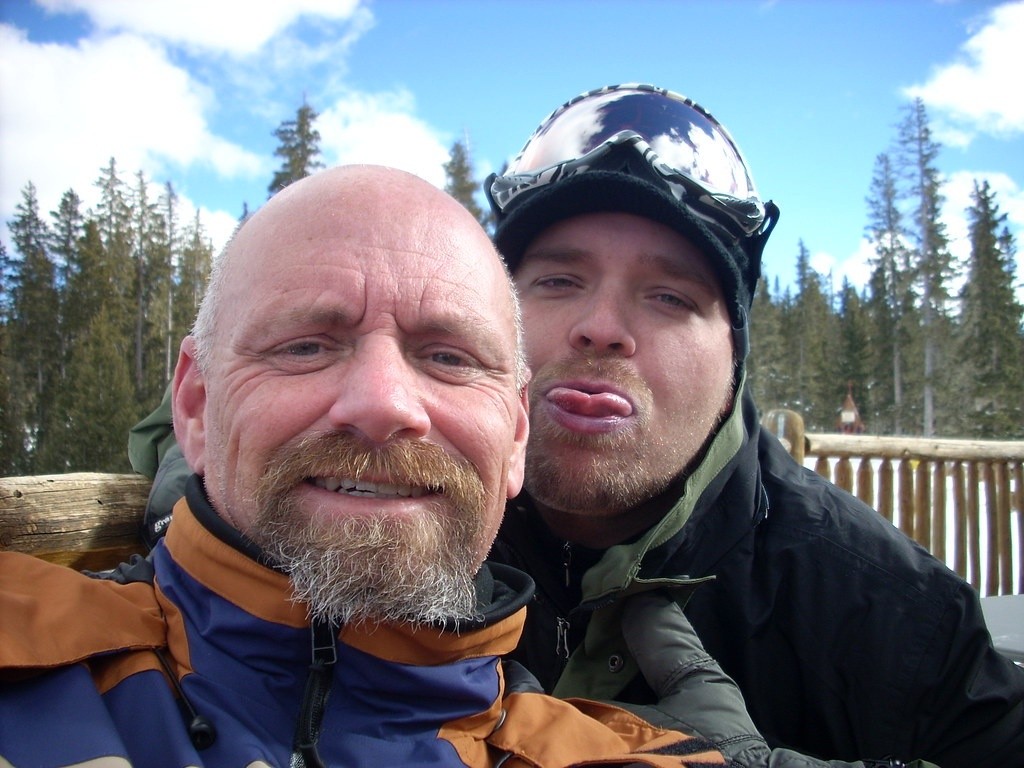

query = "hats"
[493,92,756,389]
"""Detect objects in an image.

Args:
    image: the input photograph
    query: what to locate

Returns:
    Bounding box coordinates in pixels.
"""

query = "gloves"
[595,591,867,768]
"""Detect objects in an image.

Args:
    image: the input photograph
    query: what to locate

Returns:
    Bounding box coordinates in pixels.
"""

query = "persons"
[0,162,725,768]
[126,83,1024,768]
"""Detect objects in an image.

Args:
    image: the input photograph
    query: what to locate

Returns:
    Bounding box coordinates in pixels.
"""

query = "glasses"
[490,82,766,245]
